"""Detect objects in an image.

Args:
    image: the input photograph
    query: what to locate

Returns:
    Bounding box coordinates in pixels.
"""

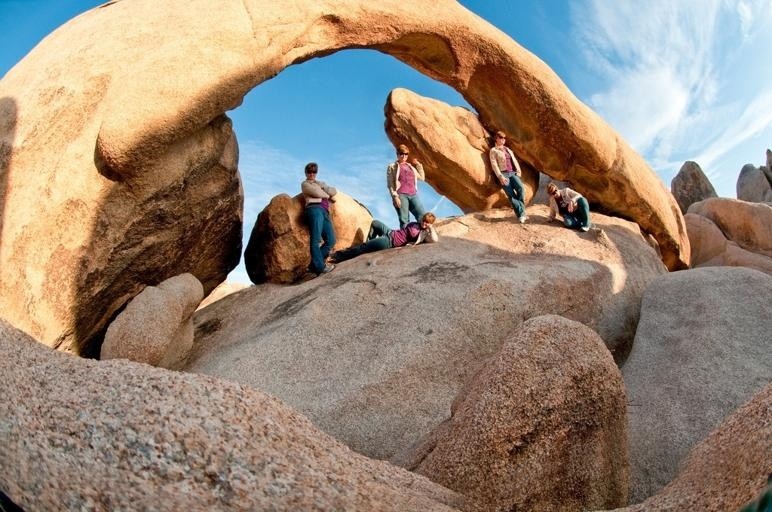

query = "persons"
[488,130,527,227]
[300,161,339,280]
[386,143,427,230]
[543,180,590,233]
[326,211,439,259]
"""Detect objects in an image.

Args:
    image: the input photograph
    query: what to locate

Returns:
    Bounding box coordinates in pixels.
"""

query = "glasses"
[307,170,316,173]
[401,153,408,155]
[499,137,506,139]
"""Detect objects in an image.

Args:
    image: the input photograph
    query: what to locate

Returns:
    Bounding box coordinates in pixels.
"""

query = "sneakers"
[319,264,335,275]
[519,216,526,223]
[579,227,590,232]
